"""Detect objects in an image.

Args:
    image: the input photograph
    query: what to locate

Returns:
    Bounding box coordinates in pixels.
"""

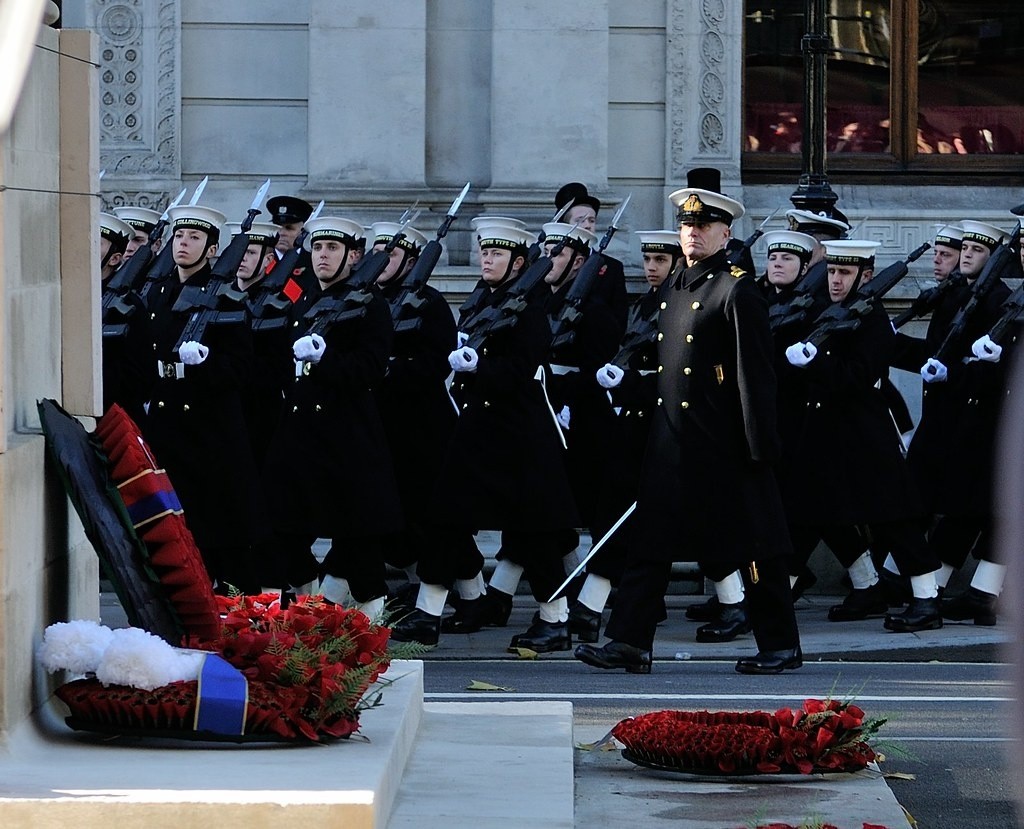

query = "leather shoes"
[509,622,572,653]
[439,613,481,634]
[735,646,803,675]
[482,589,513,627]
[530,598,602,643]
[942,586,997,626]
[884,600,943,633]
[793,566,817,603]
[827,582,889,622]
[686,594,720,622]
[389,619,440,645]
[574,639,652,674]
[696,608,752,643]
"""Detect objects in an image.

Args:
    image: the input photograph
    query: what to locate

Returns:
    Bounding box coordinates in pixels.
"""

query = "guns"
[983,278,1024,354]
[305,197,422,349]
[460,198,592,362]
[252,200,325,319]
[768,216,868,337]
[546,190,632,356]
[889,269,962,330]
[802,222,950,359]
[926,222,1022,375]
[171,178,270,359]
[102,175,209,338]
[607,205,784,379]
[386,182,472,331]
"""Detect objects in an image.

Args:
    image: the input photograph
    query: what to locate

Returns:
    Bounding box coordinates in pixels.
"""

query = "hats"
[477,225,538,258]
[820,240,881,266]
[635,230,680,254]
[471,216,528,246]
[266,195,314,224]
[166,204,228,236]
[99,212,136,245]
[761,230,818,257]
[113,206,163,233]
[784,209,849,241]
[961,219,1011,250]
[668,188,746,227]
[554,182,601,223]
[225,221,283,245]
[304,216,364,248]
[1009,203,1024,237]
[371,221,428,258]
[933,224,965,252]
[542,222,598,257]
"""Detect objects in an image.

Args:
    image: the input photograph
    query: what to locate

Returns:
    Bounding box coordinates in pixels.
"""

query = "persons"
[98,168,1024,641]
[576,186,804,677]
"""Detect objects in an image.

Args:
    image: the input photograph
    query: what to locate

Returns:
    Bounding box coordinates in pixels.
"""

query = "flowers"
[612,672,929,776]
[31,582,445,742]
[94,401,219,632]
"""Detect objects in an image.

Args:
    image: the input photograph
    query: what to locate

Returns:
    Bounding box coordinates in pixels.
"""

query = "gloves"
[971,334,1002,363]
[448,346,478,372]
[920,357,947,383]
[786,341,817,366]
[293,333,326,364]
[596,363,625,388]
[458,331,469,349]
[179,340,209,365]
[556,405,570,430]
